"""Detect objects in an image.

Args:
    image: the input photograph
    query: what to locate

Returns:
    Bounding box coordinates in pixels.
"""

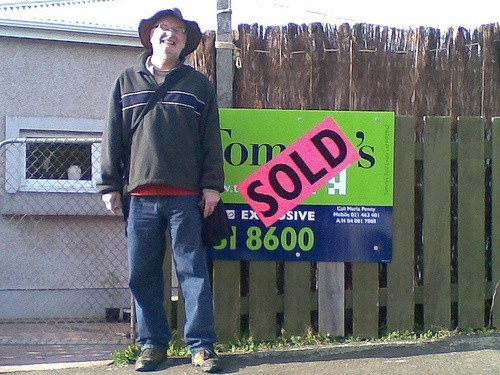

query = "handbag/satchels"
[121,169,131,237]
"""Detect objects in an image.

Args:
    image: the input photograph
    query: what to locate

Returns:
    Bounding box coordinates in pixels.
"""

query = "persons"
[96,8,225,373]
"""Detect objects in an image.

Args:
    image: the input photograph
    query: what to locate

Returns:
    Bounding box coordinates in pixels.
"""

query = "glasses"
[152,23,186,34]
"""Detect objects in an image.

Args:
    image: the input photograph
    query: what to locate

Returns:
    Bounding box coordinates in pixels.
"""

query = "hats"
[138,7,202,60]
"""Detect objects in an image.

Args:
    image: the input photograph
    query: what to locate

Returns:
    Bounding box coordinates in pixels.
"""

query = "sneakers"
[135,348,167,371]
[191,348,224,373]
